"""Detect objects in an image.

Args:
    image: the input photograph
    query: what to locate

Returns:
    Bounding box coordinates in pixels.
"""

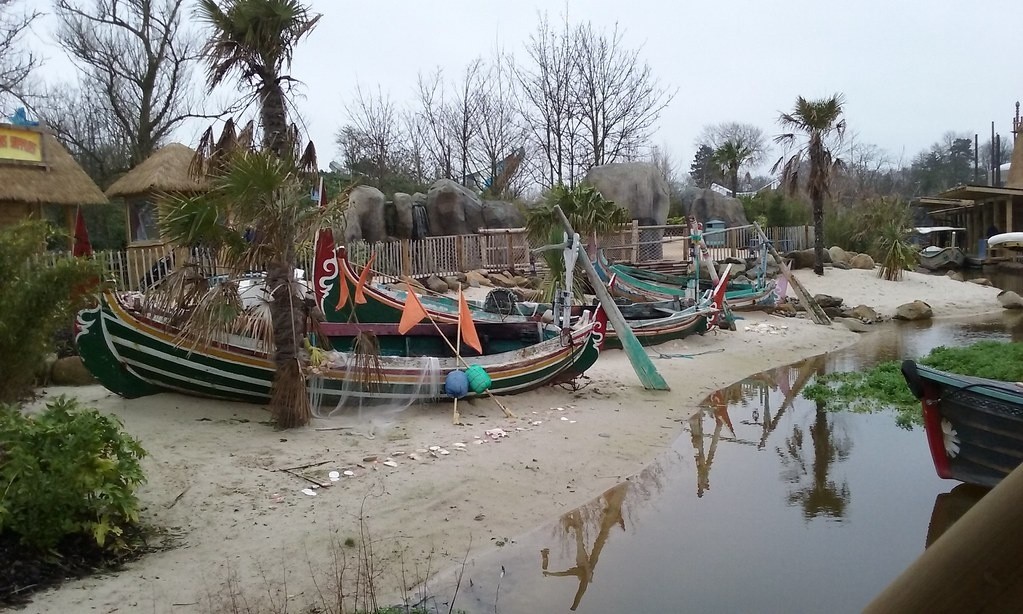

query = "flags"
[336,267,348,312]
[355,258,374,304]
[399,289,426,335]
[459,291,482,354]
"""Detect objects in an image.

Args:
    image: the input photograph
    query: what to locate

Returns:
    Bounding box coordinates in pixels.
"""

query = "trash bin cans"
[703,219,725,248]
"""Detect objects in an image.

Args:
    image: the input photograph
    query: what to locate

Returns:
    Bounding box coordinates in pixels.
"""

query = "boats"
[900,358,1023,489]
[75,269,618,407]
[589,247,777,311]
[312,228,733,349]
[908,226,967,273]
